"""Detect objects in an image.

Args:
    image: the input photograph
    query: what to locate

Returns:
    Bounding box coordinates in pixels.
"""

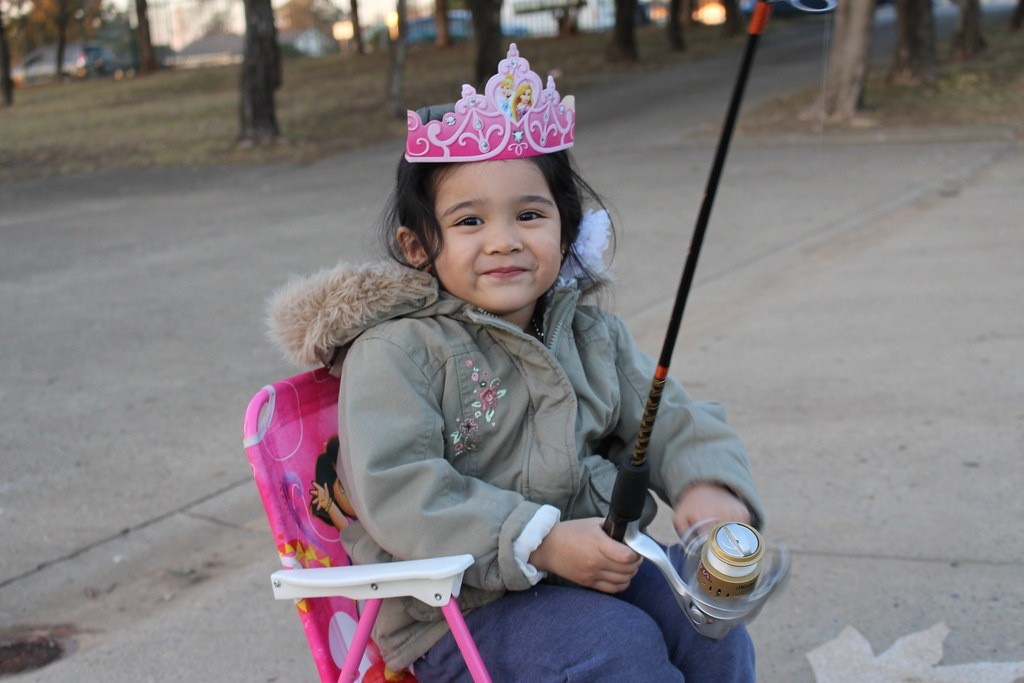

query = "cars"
[409,10,528,42]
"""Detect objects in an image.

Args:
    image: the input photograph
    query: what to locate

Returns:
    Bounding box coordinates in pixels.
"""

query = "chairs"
[242,368,494,683]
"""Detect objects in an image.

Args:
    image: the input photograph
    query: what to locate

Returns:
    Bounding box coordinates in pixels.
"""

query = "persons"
[265,42,767,683]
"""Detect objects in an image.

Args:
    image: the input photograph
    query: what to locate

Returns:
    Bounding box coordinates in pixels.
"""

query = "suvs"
[12,40,113,81]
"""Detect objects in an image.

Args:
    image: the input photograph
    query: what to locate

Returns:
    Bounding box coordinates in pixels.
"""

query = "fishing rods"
[600,0,792,645]
